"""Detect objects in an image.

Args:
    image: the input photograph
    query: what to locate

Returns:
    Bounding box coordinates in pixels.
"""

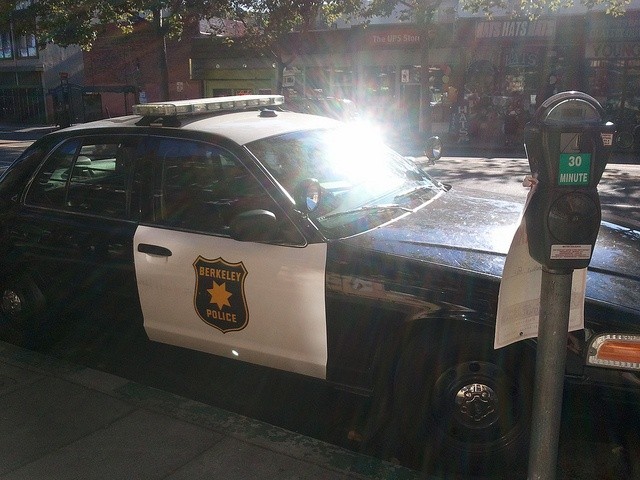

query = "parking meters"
[524,89,615,478]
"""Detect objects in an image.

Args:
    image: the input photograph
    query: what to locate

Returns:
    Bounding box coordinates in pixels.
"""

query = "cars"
[1,93,638,452]
